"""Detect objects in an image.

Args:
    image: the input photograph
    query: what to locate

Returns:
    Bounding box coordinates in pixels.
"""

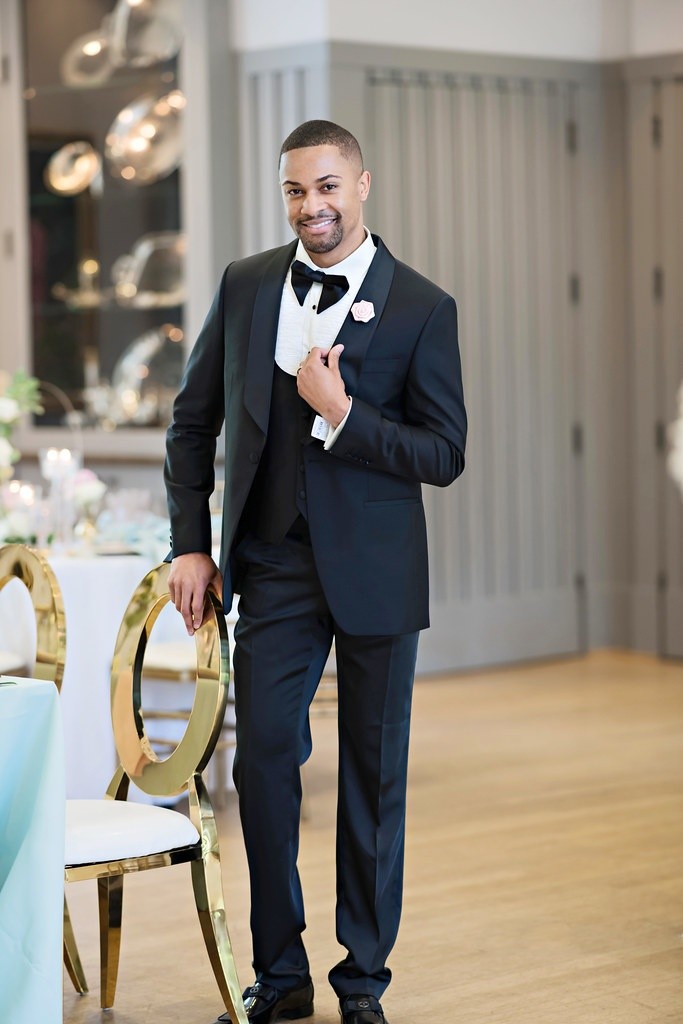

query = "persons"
[162,119,468,1024]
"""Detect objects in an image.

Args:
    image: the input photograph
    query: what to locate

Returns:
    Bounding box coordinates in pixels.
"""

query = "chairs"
[0,545,65,691]
[64,561,249,1024]
[116,619,239,819]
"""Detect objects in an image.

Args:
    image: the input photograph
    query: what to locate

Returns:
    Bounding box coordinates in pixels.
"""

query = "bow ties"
[290,260,350,314]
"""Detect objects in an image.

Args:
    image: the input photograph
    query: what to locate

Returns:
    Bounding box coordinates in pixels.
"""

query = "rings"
[297,368,301,374]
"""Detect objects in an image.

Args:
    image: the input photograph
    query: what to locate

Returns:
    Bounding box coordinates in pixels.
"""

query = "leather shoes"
[339,995,388,1024]
[216,981,314,1024]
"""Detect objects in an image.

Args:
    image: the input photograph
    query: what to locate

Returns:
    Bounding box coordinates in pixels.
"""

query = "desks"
[0,672,64,1024]
[1,517,239,812]
